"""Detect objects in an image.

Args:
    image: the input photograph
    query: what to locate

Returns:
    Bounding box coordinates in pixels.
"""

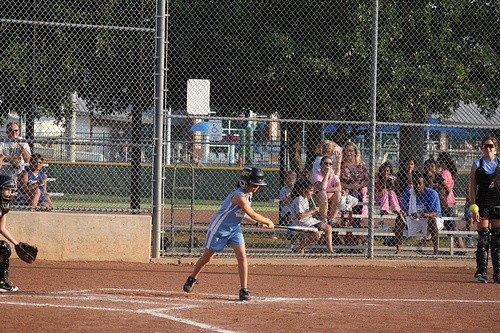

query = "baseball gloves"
[15,242,38,264]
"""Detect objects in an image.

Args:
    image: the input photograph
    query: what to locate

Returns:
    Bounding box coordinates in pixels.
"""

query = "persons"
[0,174,19,293]
[310,155,342,230]
[183,167,275,302]
[0,122,31,197]
[393,175,441,260]
[291,177,335,254]
[23,153,54,211]
[471,137,500,283]
[278,138,468,255]
[464,177,479,248]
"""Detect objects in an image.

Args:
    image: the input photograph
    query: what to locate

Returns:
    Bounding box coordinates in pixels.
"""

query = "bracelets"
[421,212,424,217]
[318,189,320,191]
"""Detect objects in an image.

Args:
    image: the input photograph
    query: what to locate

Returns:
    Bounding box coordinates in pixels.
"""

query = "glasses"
[32,161,41,164]
[8,129,18,132]
[482,143,494,149]
[324,162,333,166]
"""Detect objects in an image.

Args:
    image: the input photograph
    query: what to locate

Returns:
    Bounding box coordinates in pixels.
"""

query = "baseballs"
[469,204,479,213]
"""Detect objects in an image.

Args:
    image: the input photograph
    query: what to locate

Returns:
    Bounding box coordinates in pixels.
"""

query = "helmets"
[241,166,267,186]
[0,174,18,214]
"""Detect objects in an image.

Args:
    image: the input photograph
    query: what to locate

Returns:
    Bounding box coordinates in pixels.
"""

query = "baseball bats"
[255,221,319,233]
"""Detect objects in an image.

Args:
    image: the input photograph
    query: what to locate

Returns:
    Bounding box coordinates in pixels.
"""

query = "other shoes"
[416,250,424,254]
[329,220,339,227]
[475,273,489,284]
[467,236,475,248]
[296,245,304,255]
[326,249,336,254]
[393,211,404,215]
[453,251,469,256]
[493,276,500,283]
[395,245,401,254]
[380,210,388,215]
[0,282,18,293]
[322,219,328,225]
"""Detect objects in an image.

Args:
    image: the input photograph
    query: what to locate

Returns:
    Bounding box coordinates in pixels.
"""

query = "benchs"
[273,195,491,255]
[2,162,65,197]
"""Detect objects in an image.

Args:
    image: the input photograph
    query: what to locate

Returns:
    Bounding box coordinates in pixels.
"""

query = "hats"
[30,153,44,161]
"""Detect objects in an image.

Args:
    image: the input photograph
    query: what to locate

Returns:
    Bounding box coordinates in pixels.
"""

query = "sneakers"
[239,289,250,300]
[183,276,197,293]
